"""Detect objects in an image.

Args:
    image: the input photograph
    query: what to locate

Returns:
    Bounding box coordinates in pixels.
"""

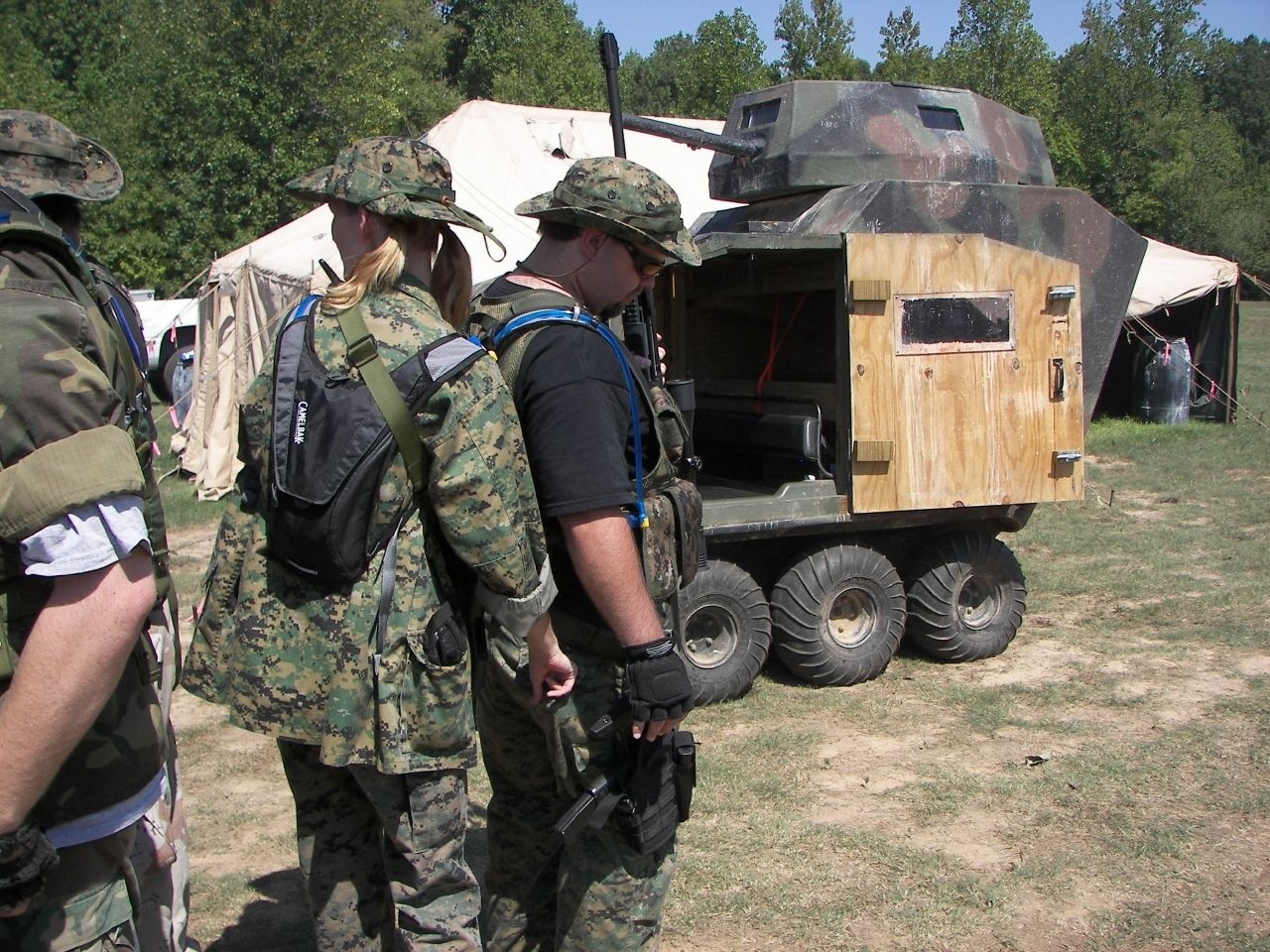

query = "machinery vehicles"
[599,33,1148,706]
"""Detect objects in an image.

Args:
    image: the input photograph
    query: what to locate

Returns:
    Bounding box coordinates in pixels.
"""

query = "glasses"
[619,238,665,281]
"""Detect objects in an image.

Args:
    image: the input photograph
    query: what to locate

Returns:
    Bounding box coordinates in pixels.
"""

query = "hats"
[514,156,703,267]
[0,109,124,202]
[282,135,493,233]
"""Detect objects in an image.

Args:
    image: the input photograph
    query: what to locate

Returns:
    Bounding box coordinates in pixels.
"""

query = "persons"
[183,133,574,952]
[0,108,194,952]
[477,154,699,951]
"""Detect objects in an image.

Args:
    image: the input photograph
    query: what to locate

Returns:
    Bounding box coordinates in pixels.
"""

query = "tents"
[177,97,1241,499]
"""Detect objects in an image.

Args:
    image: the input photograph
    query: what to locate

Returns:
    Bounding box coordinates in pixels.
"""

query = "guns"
[597,32,710,572]
[672,731,697,822]
[555,696,676,864]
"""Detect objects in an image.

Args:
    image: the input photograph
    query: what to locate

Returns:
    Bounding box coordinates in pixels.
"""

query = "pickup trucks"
[131,299,201,402]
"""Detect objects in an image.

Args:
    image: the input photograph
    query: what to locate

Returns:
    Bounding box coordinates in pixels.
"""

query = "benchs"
[697,393,835,478]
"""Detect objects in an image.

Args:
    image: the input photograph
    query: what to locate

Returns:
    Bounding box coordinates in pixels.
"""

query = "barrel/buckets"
[1132,333,1190,425]
